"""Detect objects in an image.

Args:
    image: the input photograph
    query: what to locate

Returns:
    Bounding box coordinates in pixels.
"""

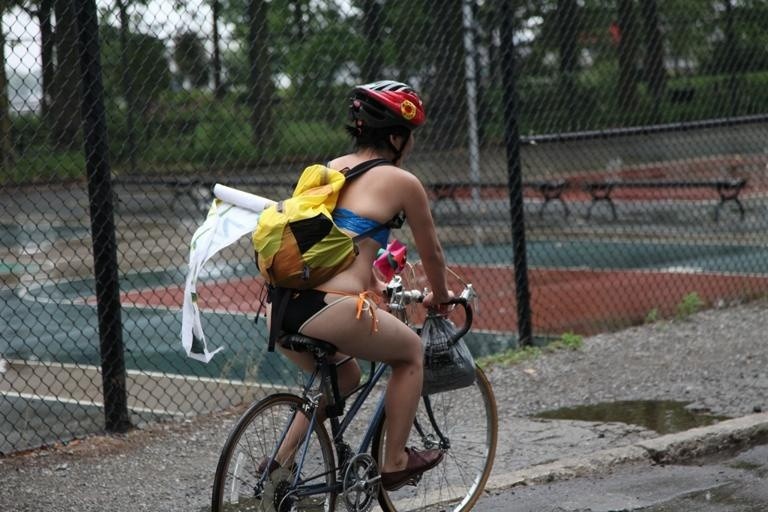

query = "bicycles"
[210,275,499,512]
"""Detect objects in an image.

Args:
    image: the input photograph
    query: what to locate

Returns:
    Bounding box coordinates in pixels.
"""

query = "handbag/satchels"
[422,317,476,394]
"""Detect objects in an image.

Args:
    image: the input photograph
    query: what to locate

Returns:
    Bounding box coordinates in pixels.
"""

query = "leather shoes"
[381,447,442,491]
[258,455,304,485]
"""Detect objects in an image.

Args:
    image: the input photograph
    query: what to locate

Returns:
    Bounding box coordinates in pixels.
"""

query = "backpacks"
[255,158,398,291]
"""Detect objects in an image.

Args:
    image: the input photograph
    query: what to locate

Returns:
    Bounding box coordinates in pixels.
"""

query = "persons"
[253,80,455,501]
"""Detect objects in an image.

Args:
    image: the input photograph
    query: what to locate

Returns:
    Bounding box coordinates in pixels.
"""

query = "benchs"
[110,172,750,223]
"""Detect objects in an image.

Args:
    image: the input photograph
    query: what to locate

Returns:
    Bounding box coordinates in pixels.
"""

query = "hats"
[348,80,425,127]
